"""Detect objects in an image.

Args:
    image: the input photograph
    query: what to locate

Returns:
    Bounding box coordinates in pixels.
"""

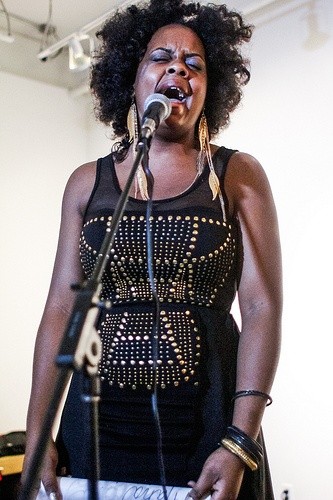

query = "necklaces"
[171,173,198,197]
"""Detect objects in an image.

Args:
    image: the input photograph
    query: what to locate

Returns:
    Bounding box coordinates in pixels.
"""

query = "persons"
[20,0,283,500]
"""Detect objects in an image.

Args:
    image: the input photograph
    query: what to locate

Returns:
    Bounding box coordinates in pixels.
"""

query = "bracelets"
[221,424,264,471]
[231,388,273,407]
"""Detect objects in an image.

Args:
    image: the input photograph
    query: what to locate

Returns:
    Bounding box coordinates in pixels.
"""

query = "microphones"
[135,92,172,153]
